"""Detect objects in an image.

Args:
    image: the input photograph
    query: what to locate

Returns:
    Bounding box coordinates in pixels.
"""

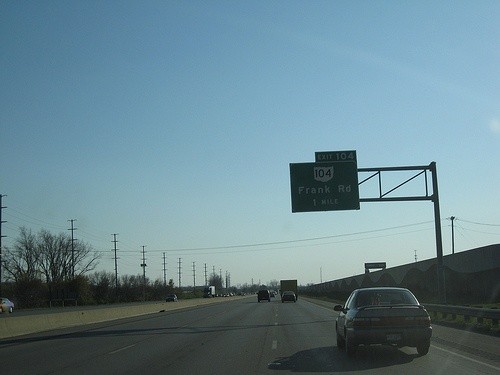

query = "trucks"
[279,280,298,301]
[201,286,215,298]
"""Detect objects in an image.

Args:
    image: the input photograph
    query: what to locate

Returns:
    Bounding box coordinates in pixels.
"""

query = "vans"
[256,289,271,303]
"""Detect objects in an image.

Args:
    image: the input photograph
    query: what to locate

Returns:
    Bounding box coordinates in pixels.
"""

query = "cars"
[281,290,296,303]
[333,286,433,357]
[0,298,15,313]
[269,290,278,297]
[166,294,178,302]
